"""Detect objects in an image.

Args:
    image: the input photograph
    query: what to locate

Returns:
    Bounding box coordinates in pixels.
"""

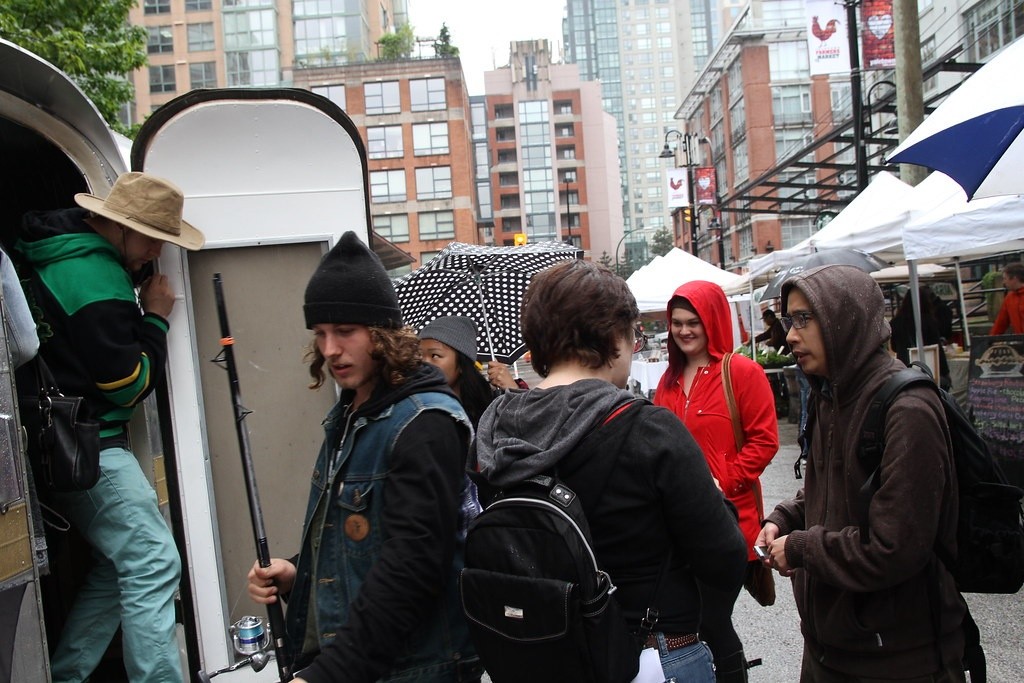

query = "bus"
[0,36,375,683]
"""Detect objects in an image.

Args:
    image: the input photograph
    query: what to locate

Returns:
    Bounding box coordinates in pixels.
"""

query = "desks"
[629,360,669,401]
[945,349,968,412]
[763,368,789,420]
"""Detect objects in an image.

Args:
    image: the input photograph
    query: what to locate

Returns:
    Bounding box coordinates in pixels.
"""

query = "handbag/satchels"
[26,350,101,494]
[742,521,776,607]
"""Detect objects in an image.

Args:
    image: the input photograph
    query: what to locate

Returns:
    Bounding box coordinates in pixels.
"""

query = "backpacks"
[457,472,692,683]
[794,356,1023,593]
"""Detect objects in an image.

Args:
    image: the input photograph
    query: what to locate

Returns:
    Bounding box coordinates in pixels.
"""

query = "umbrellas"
[390,240,587,394]
[884,34,1024,202]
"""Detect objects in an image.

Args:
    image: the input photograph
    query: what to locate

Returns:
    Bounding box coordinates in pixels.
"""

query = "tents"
[623,169,1024,360]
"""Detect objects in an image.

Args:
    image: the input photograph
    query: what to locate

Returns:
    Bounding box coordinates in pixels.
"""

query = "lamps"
[751,247,756,252]
[729,256,735,259]
[765,244,775,251]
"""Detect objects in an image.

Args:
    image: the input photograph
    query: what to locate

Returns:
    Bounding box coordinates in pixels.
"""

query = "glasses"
[781,311,814,333]
[630,325,648,354]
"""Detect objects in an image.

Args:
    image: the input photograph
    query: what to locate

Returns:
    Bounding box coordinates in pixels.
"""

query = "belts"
[640,628,699,653]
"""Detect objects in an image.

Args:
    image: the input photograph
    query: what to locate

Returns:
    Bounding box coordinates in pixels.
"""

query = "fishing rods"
[193,271,293,683]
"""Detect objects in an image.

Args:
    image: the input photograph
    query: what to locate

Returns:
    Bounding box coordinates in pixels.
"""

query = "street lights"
[658,130,698,256]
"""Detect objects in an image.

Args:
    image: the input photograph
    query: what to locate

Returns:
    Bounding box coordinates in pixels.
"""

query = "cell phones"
[753,546,771,559]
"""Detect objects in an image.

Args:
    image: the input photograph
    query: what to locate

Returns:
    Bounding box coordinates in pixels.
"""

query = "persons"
[247,230,479,683]
[888,288,951,391]
[989,260,1024,334]
[743,309,791,355]
[418,316,529,506]
[11,171,206,683]
[653,280,779,683]
[467,258,749,683]
[923,286,954,345]
[755,264,966,683]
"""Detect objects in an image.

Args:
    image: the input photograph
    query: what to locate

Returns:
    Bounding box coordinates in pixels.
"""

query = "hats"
[302,229,404,329]
[759,309,775,319]
[419,315,479,365]
[74,171,206,251]
